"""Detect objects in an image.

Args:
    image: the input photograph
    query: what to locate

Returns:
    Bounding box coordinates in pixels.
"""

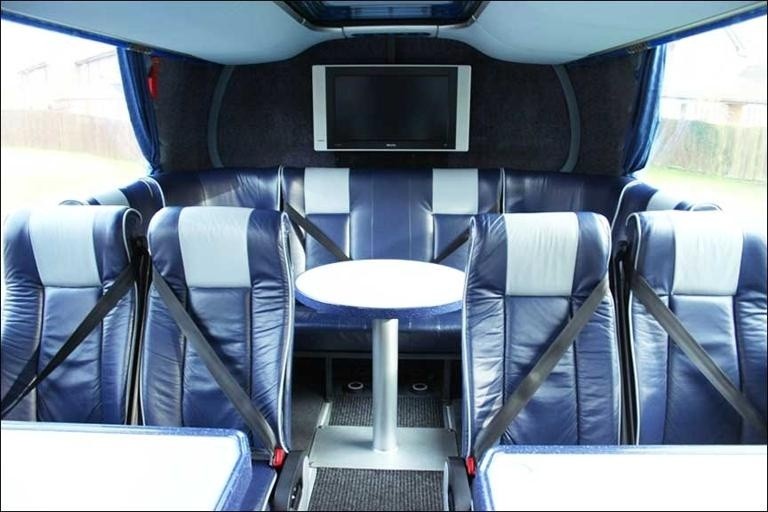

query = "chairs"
[472,441,768,512]
[0,200,144,427]
[133,205,312,512]
[55,166,721,334]
[620,210,767,447]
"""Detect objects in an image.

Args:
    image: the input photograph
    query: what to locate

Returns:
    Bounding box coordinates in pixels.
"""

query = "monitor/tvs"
[311,65,472,153]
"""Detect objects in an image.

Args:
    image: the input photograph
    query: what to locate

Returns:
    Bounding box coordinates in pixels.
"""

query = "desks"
[440,210,630,511]
[0,418,255,511]
[294,258,466,474]
